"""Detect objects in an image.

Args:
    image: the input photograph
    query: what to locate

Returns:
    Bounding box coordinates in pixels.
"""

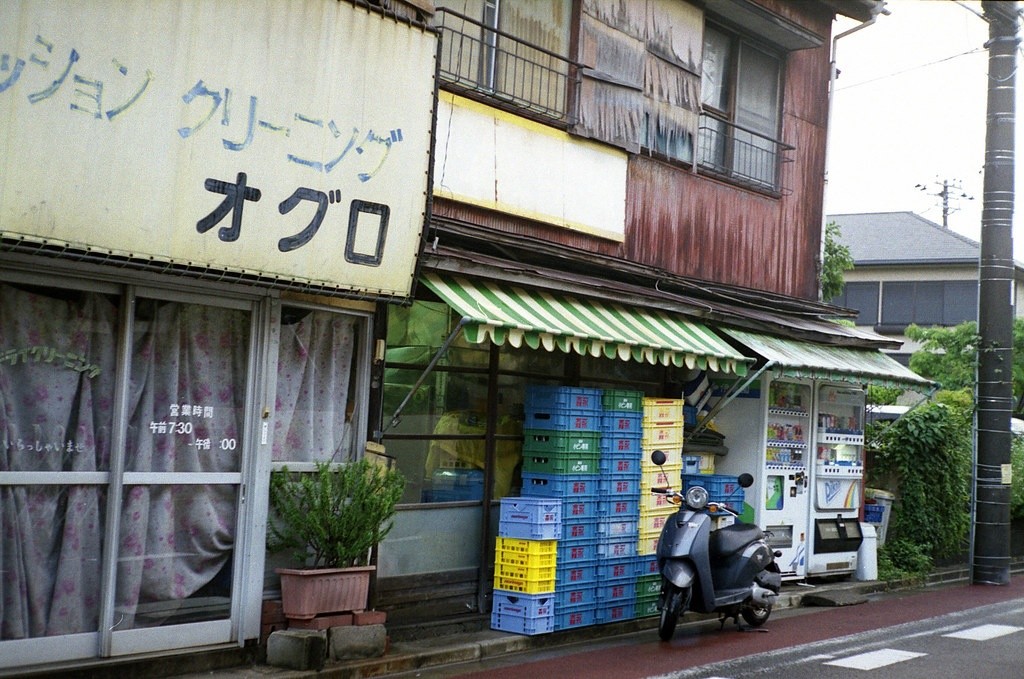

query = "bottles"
[767,382,806,465]
[817,412,861,466]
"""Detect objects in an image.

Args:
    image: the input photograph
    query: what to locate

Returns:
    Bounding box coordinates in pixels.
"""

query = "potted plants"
[266,458,403,620]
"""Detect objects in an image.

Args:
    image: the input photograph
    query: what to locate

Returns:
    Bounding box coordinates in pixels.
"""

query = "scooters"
[649,447,783,641]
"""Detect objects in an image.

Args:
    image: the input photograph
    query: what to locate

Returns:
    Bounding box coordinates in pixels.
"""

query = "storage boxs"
[492,388,745,634]
[439,448,478,470]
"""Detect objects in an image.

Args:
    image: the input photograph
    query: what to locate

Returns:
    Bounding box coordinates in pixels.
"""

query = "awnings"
[379,270,758,444]
[689,328,942,449]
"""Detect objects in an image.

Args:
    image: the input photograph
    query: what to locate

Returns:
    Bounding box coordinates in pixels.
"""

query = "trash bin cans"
[849,522,879,581]
[864,487,896,549]
